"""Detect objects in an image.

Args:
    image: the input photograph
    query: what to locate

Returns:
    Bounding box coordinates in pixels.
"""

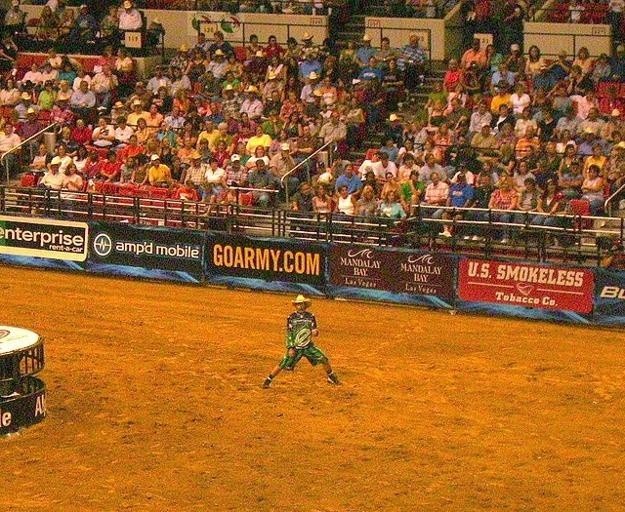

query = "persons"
[1,1,625,272]
[262,293,342,390]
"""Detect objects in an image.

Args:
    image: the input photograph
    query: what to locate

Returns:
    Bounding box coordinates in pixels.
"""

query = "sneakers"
[327,377,342,386]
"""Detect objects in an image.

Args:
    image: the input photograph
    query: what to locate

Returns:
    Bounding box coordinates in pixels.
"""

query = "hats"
[613,141,625,150]
[292,295,312,307]
[390,114,401,122]
[47,157,61,169]
[281,143,290,151]
[12,0,372,118]
[231,154,240,163]
[151,154,160,161]
[581,127,595,137]
[511,44,520,52]
